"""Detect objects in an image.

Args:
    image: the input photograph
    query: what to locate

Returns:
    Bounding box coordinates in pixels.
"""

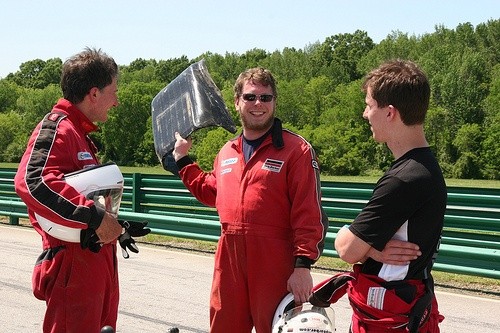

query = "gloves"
[80,218,150,258]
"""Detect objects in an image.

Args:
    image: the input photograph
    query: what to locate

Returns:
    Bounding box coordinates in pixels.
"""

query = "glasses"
[237,94,277,102]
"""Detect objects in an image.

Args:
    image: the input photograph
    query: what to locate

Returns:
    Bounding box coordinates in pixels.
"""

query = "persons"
[14,47,123,333]
[333,58,449,332]
[169,68,329,333]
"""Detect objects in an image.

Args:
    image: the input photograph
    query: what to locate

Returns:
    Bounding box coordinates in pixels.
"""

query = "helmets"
[271,292,338,333]
[34,162,125,245]
